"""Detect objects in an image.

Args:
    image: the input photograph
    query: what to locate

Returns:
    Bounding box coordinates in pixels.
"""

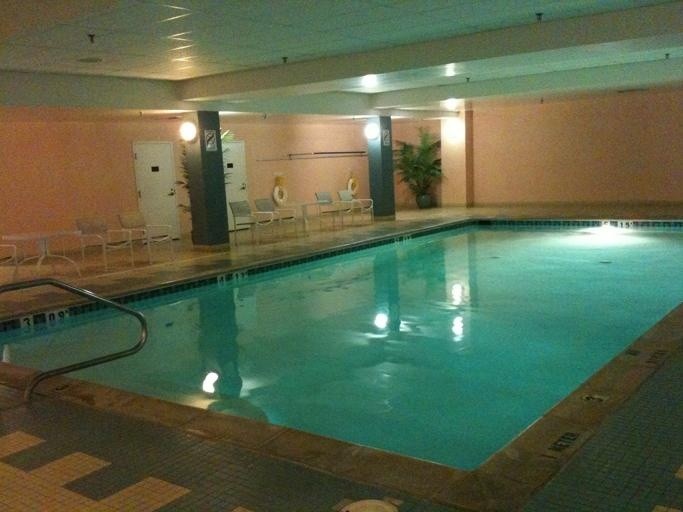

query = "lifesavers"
[348,178,360,196]
[273,186,288,206]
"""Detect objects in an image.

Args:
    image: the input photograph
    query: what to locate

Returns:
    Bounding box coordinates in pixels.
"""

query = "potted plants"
[391,132,441,208]
